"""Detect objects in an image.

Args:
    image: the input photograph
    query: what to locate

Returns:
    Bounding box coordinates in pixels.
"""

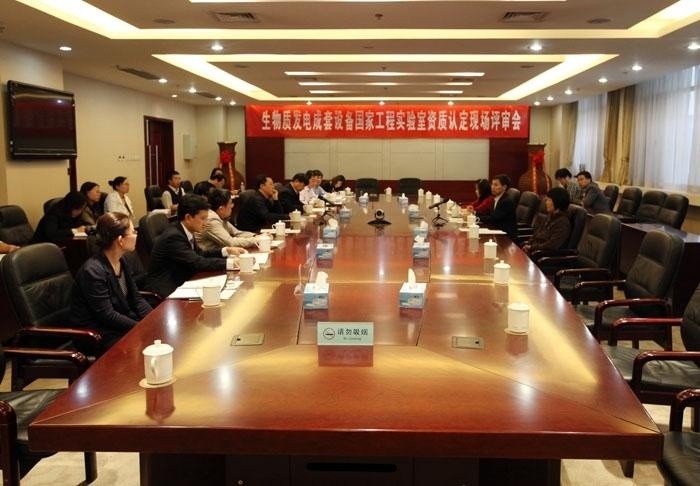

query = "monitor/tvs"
[7,79,77,160]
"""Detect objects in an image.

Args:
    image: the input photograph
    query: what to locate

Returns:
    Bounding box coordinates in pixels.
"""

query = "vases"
[216,142,245,190]
[518,143,553,196]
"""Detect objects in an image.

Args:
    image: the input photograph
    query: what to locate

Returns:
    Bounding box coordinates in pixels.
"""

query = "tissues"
[399,193,409,204]
[398,268,427,309]
[412,235,430,259]
[414,221,428,240]
[407,204,419,218]
[359,192,368,203]
[323,218,339,238]
[303,271,330,310]
[317,243,333,259]
[340,206,352,218]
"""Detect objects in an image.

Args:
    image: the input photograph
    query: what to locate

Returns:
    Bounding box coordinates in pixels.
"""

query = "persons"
[520,187,572,271]
[555,168,582,206]
[1,242,21,259]
[463,177,496,216]
[23,167,348,347]
[576,171,612,212]
[474,174,518,238]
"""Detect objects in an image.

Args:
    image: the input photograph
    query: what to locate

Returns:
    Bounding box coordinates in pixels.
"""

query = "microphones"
[318,194,335,206]
[429,197,449,208]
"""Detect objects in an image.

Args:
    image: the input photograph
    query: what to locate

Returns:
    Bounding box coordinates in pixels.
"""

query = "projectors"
[367,208,392,224]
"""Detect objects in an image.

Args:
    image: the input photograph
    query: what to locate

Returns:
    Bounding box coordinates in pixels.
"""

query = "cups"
[255,235,272,251]
[469,223,480,238]
[483,238,497,258]
[507,299,529,332]
[237,251,257,274]
[303,186,354,214]
[195,282,222,306]
[144,336,176,385]
[272,218,286,234]
[493,258,511,283]
[384,186,392,194]
[419,187,462,217]
[289,208,302,220]
[466,212,481,226]
